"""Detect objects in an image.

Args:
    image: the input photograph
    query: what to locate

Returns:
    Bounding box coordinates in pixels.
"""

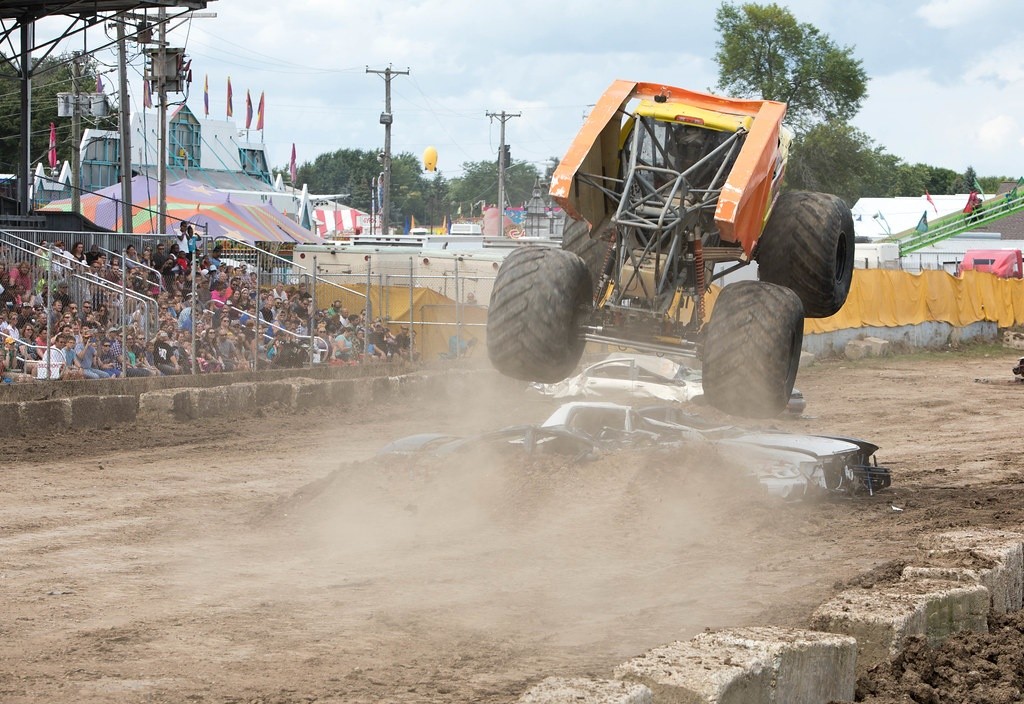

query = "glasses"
[277,301,283,305]
[140,338,145,340]
[37,309,43,311]
[159,247,165,250]
[224,320,230,322]
[60,341,66,344]
[104,344,111,347]
[209,332,216,334]
[232,283,238,286]
[85,306,92,308]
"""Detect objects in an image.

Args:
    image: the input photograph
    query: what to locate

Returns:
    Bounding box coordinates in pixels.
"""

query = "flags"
[204,74,209,114]
[95,72,102,94]
[915,177,1024,232]
[290,143,297,186]
[246,91,253,128]
[227,76,232,116]
[257,92,264,129]
[144,66,152,107]
[48,123,57,168]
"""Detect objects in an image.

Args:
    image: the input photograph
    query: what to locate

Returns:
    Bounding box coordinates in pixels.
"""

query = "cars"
[535,400,895,505]
[376,424,676,478]
[527,354,806,415]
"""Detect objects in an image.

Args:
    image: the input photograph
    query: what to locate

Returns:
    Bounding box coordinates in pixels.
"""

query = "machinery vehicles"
[484,79,857,419]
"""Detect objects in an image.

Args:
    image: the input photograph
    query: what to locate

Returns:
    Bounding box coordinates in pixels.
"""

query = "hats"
[60,282,67,287]
[6,337,16,344]
[109,327,119,333]
[210,265,216,271]
[22,302,31,308]
[240,261,247,267]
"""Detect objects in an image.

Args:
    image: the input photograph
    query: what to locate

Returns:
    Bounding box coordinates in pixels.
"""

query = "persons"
[669,125,719,188]
[0,222,420,385]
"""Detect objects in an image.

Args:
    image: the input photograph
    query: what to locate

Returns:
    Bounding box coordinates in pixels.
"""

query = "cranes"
[854,183,1024,278]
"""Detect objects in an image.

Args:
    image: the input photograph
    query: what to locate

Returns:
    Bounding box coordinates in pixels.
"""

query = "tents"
[282,185,377,237]
[265,281,489,371]
